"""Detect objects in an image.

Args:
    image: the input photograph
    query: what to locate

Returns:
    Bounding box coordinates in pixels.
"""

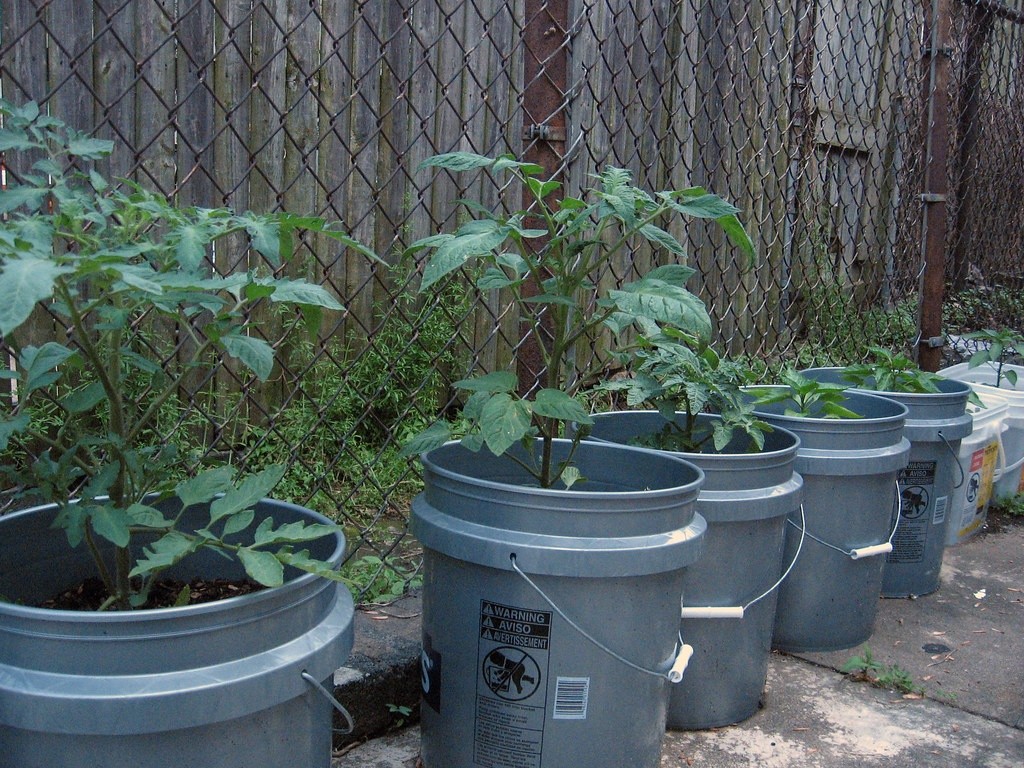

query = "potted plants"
[736,364,913,654]
[946,389,1010,549]
[937,325,1024,503]
[415,154,758,768]
[0,95,401,768]
[795,344,974,597]
[586,324,808,731]
